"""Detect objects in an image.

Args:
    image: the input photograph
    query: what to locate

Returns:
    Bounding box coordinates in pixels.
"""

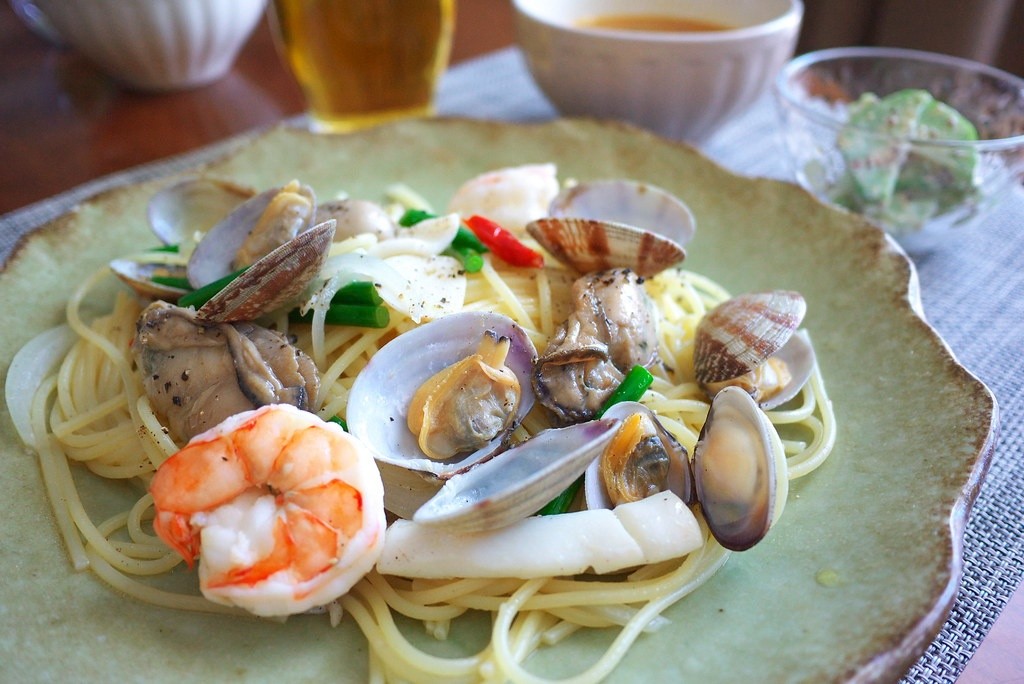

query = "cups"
[274,0,457,132]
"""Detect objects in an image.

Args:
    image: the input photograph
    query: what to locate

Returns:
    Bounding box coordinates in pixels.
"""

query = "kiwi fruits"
[838,88,982,210]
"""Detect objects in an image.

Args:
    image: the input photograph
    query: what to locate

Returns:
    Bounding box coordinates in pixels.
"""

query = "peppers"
[463,214,543,268]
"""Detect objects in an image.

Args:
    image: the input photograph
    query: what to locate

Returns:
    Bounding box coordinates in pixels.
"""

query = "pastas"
[31,184,837,684]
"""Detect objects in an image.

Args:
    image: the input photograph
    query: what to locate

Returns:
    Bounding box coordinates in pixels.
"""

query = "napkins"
[0,49,1024,684]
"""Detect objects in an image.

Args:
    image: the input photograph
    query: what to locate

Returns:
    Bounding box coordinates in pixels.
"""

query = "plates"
[1,120,1000,684]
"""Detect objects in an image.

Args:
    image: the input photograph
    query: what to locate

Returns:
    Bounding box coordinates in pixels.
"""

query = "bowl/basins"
[777,47,1024,250]
[512,1,804,146]
[32,0,269,92]
